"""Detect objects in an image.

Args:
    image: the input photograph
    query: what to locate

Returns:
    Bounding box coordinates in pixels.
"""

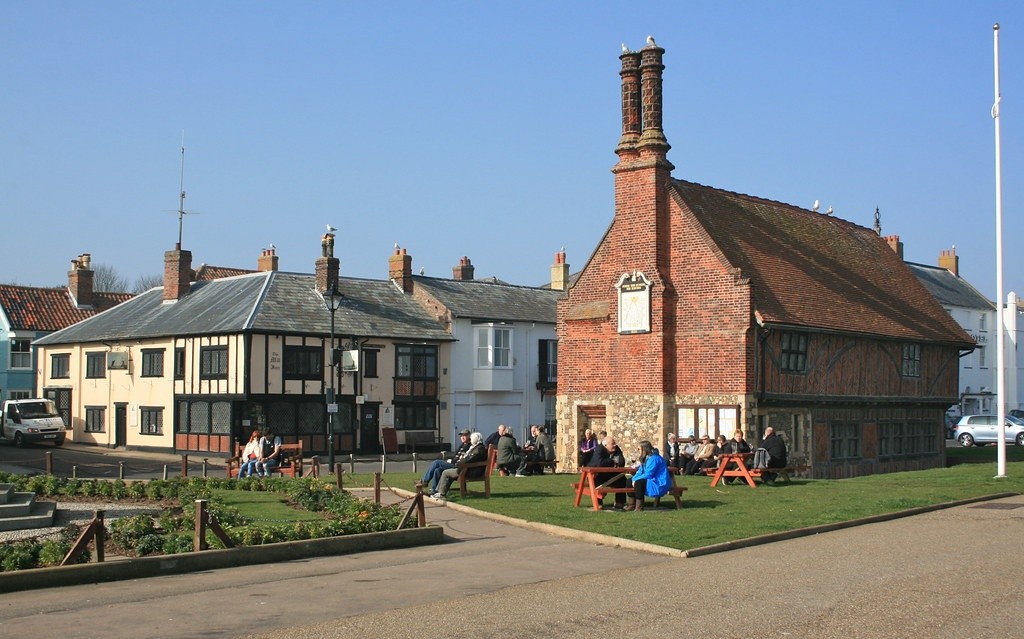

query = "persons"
[485,424,556,478]
[237,427,281,480]
[759,426,786,483]
[722,429,750,485]
[588,436,627,509]
[666,431,729,476]
[580,428,607,466]
[416,428,485,500]
[627,441,673,512]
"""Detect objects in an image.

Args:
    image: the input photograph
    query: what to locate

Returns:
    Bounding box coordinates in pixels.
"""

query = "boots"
[633,499,644,512]
[624,497,637,511]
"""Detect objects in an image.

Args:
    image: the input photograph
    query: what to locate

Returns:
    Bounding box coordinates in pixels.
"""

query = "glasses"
[691,440,695,441]
[702,439,706,441]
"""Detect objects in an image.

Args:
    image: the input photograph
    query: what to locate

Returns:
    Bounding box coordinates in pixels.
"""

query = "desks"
[573,467,684,513]
[489,449,556,477]
[710,452,791,489]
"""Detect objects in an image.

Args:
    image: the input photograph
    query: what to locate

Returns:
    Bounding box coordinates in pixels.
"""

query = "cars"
[945,408,1024,448]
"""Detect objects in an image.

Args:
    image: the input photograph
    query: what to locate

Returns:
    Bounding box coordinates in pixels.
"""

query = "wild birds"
[812,200,819,212]
[825,205,833,215]
[560,246,567,252]
[269,242,276,249]
[419,267,425,276]
[325,223,338,233]
[394,242,401,249]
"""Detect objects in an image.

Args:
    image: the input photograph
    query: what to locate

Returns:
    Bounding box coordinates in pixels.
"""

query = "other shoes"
[598,504,603,508]
[722,477,729,485]
[429,493,447,500]
[424,491,434,496]
[613,503,626,510]
[768,480,774,485]
[414,479,428,487]
[515,474,526,477]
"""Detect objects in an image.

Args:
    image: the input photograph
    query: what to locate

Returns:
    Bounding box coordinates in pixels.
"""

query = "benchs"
[594,487,688,499]
[748,465,812,477]
[702,467,718,477]
[527,460,559,469]
[225,440,304,478]
[454,443,495,499]
[569,482,589,494]
[404,431,444,454]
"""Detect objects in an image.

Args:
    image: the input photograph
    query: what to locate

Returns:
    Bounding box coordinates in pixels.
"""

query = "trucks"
[0,398,66,448]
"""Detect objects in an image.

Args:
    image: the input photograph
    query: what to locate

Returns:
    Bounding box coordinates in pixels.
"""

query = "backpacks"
[754,448,771,470]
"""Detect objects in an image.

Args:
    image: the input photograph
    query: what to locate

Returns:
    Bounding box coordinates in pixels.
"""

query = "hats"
[458,429,470,434]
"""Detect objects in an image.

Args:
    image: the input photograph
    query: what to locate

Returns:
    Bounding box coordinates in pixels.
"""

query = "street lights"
[322,280,344,476]
